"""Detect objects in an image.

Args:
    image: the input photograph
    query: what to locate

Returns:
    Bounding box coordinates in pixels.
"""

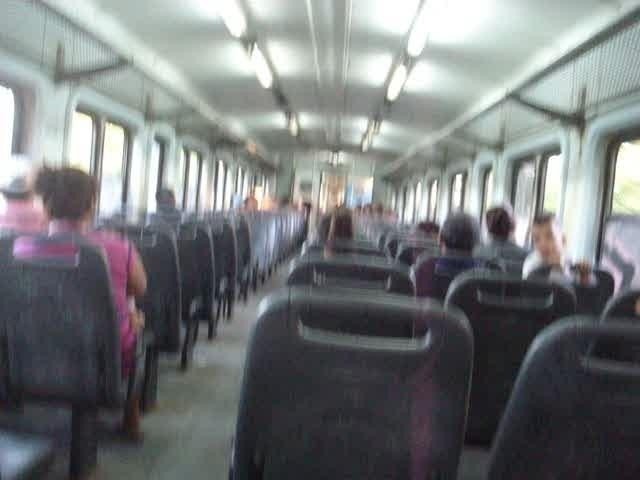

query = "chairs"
[227,205,639,480]
[1,204,306,480]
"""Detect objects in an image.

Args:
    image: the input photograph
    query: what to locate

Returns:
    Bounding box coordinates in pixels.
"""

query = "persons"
[437,205,595,289]
[13,165,149,447]
[0,154,51,233]
[155,190,401,257]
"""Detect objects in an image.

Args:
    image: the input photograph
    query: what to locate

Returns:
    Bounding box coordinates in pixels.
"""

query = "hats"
[0,156,35,193]
